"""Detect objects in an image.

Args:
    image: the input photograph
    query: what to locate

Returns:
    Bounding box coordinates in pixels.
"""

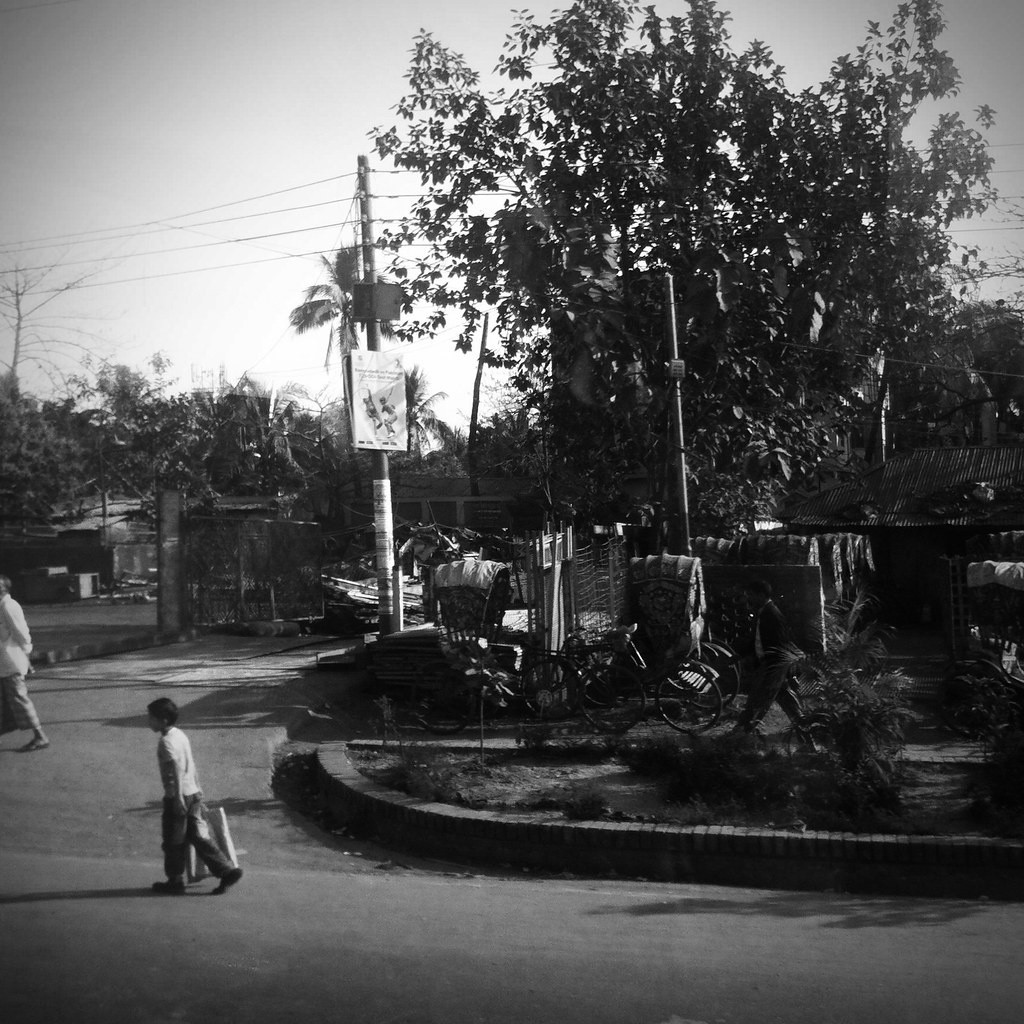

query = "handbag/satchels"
[185,804,239,884]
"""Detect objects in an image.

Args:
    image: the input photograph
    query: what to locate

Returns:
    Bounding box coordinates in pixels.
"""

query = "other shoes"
[212,867,242,895]
[153,879,184,894]
[20,740,50,752]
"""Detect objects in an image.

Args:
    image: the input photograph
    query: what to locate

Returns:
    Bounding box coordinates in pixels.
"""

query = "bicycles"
[518,619,741,734]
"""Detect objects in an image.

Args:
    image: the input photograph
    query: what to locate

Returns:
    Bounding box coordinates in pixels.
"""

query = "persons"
[729,581,819,745]
[146,698,244,895]
[0,574,50,752]
